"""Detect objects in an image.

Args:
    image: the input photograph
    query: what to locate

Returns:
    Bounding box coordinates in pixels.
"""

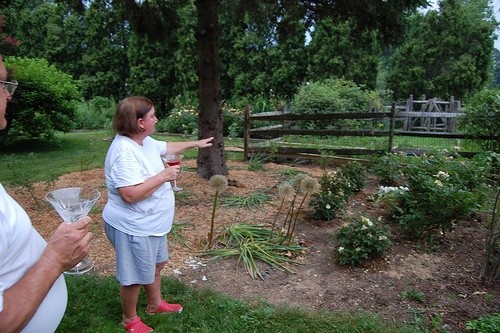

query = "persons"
[0,56,93,333]
[104,96,214,333]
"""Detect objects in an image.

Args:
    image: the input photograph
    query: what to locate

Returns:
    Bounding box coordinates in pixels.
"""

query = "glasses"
[0,79,18,96]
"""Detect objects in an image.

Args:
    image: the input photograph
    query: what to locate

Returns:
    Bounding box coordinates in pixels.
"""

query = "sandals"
[145,300,182,315]
[122,316,153,333]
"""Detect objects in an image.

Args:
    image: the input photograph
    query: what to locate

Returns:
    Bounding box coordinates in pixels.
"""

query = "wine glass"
[46,186,102,275]
[162,154,185,192]
